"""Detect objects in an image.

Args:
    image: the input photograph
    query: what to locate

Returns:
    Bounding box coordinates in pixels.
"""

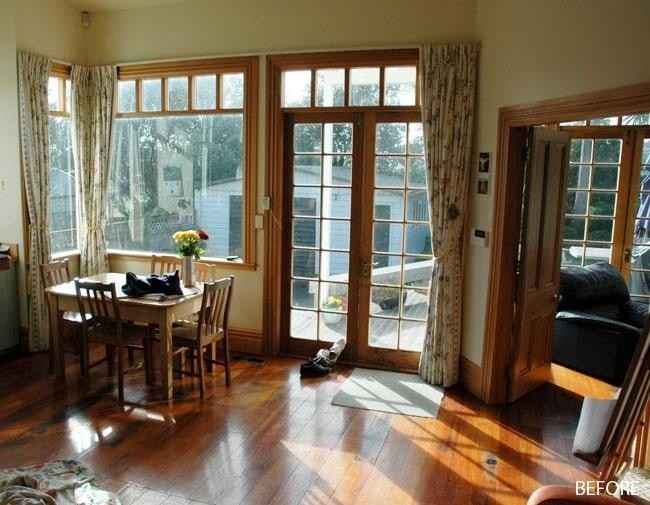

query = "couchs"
[542,260,650,458]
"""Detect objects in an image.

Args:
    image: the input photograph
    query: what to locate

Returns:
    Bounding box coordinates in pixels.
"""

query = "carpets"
[331,366,448,419]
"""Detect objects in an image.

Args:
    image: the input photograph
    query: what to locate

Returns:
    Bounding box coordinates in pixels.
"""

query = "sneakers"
[330,338,346,361]
[306,357,333,366]
[317,347,329,358]
[299,363,331,377]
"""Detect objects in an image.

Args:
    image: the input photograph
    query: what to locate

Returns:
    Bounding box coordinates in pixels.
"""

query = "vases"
[181,254,193,288]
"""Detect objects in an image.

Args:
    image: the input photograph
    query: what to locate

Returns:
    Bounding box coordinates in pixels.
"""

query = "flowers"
[171,227,210,259]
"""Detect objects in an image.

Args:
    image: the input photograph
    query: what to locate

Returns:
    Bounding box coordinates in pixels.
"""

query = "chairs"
[37,256,108,379]
[170,273,237,404]
[143,253,183,275]
[191,261,218,284]
[70,274,151,408]
